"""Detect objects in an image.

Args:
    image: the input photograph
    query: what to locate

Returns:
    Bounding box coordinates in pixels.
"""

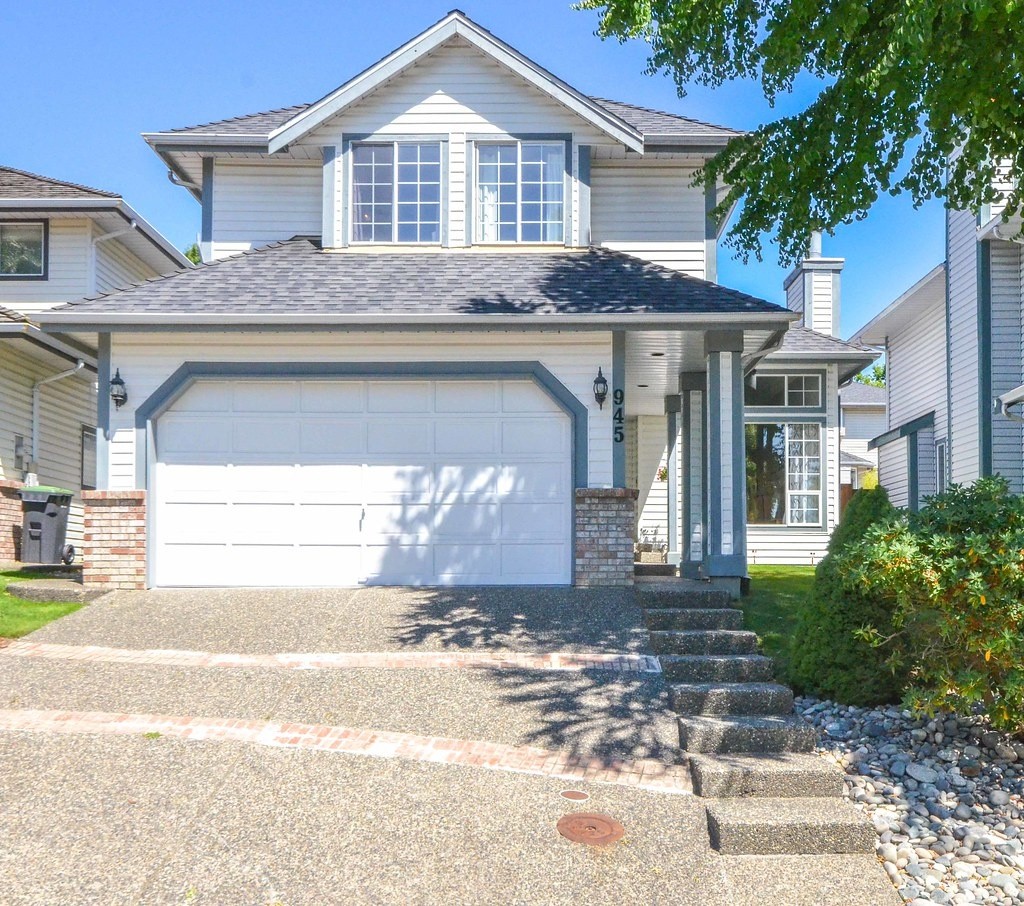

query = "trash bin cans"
[17,485,75,564]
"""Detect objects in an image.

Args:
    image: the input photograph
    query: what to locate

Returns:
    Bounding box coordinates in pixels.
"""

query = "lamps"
[109,368,128,410]
[593,367,608,409]
[744,369,757,390]
